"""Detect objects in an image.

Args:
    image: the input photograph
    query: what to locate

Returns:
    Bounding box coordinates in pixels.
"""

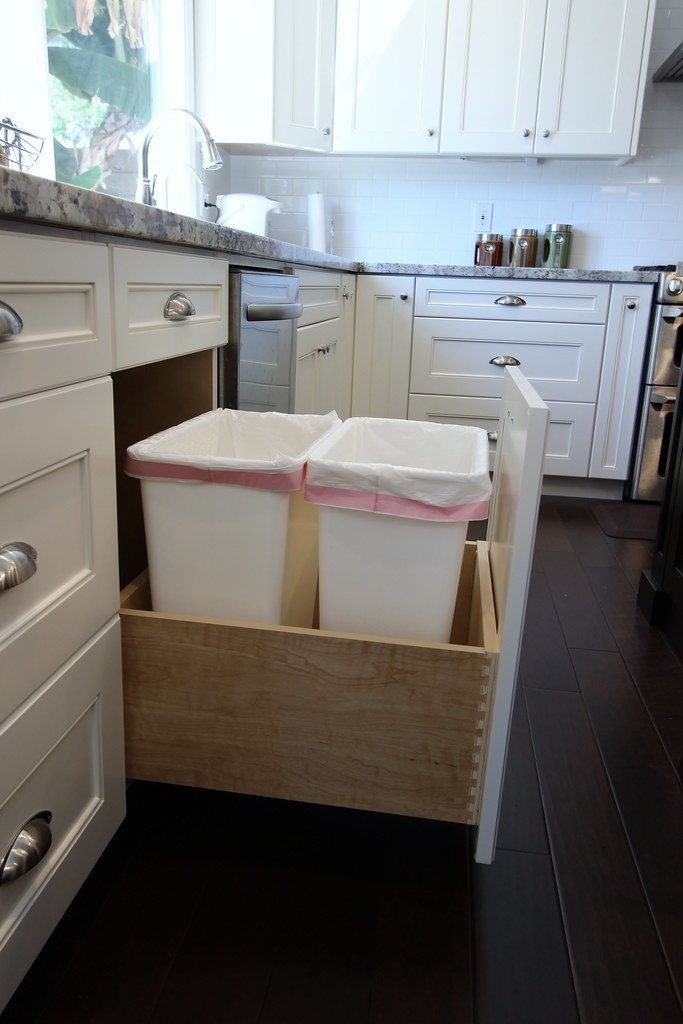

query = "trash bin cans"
[127,404,495,649]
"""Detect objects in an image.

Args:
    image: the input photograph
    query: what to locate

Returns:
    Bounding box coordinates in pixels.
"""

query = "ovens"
[628,272,683,503]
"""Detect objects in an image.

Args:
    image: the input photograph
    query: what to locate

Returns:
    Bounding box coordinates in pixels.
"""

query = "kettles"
[217,193,279,236]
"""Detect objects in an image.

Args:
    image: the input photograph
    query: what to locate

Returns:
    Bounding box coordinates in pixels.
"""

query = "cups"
[474,233,504,266]
[540,224,573,268]
[506,228,538,268]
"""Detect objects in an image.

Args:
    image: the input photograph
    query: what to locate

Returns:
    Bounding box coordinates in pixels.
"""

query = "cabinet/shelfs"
[408,276,612,477]
[438,0,649,157]
[195,0,337,155]
[0,230,552,1015]
[332,0,449,157]
[286,266,357,421]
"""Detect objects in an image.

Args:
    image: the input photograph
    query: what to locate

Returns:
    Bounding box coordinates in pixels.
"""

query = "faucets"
[134,109,223,206]
